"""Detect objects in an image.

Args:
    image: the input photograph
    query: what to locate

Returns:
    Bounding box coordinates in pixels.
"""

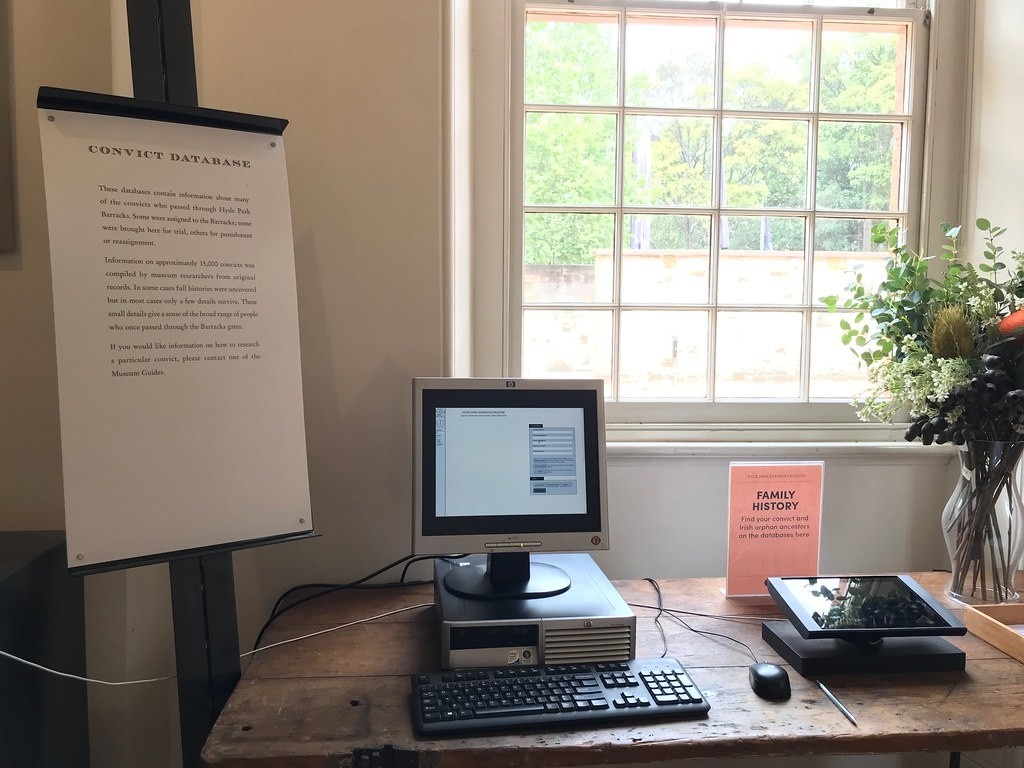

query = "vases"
[941,439,1024,606]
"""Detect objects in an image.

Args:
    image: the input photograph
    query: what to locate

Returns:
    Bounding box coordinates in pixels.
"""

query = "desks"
[200,570,1024,768]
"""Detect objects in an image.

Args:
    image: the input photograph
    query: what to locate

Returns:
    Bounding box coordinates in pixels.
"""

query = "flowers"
[818,218,1024,604]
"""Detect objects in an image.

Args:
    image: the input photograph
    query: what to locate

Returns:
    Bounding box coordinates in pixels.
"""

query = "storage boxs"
[964,603,1024,664]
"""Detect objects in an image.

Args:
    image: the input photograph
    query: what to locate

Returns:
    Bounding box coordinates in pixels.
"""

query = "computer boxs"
[431,552,636,673]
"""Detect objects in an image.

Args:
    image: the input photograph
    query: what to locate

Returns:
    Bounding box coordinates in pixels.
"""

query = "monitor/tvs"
[765,575,967,640]
[411,377,609,601]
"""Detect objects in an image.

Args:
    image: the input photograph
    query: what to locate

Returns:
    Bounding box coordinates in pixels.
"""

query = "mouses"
[749,661,792,702]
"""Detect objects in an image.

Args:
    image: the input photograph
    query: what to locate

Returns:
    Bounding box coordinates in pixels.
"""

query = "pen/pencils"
[815,679,858,725]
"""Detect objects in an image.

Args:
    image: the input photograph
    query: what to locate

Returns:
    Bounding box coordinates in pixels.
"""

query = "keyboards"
[412,656,710,737]
